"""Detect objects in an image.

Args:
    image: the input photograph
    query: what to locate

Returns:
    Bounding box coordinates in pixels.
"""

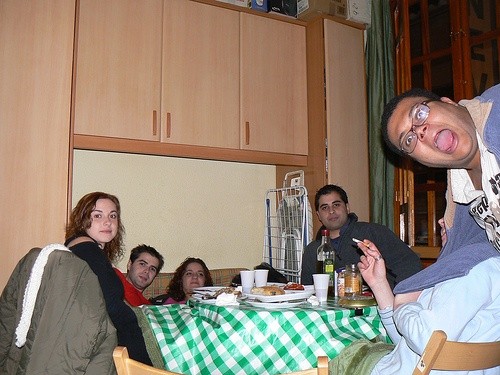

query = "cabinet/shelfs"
[0,0,369,294]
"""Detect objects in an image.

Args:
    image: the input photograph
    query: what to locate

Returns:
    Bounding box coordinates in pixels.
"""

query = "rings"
[375,256,382,261]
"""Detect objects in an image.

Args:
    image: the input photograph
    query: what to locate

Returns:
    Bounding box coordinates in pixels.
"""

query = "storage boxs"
[218,0,247,8]
[297,0,348,20]
[268,0,298,20]
[346,0,372,28]
[248,0,267,13]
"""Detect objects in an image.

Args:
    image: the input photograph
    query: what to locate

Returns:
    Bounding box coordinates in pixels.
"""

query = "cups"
[254,270,269,288]
[312,274,330,302]
[239,270,255,291]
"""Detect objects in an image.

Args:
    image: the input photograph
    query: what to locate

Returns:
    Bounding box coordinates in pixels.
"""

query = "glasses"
[399,97,440,155]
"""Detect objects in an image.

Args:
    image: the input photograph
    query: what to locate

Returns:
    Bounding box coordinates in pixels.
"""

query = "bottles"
[336,266,346,297]
[344,264,362,297]
[317,230,335,300]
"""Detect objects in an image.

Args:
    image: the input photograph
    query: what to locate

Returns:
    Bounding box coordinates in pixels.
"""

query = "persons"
[380,84,500,294]
[63,191,153,367]
[114,244,164,306]
[164,257,213,304]
[301,184,422,289]
[329,218,500,375]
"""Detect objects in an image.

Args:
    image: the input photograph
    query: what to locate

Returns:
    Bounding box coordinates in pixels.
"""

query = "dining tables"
[130,298,394,375]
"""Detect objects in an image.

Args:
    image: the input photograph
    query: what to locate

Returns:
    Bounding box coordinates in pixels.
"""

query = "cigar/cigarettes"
[352,238,369,247]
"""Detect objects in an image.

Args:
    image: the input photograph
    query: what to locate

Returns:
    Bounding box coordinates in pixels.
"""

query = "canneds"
[344,263,362,298]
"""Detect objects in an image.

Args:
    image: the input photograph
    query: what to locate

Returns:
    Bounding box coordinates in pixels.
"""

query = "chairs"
[19,244,500,375]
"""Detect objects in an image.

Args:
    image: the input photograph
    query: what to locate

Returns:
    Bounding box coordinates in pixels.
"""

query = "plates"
[192,287,241,304]
[242,283,315,308]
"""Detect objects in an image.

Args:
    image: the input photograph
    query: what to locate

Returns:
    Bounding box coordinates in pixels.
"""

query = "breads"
[252,284,285,296]
